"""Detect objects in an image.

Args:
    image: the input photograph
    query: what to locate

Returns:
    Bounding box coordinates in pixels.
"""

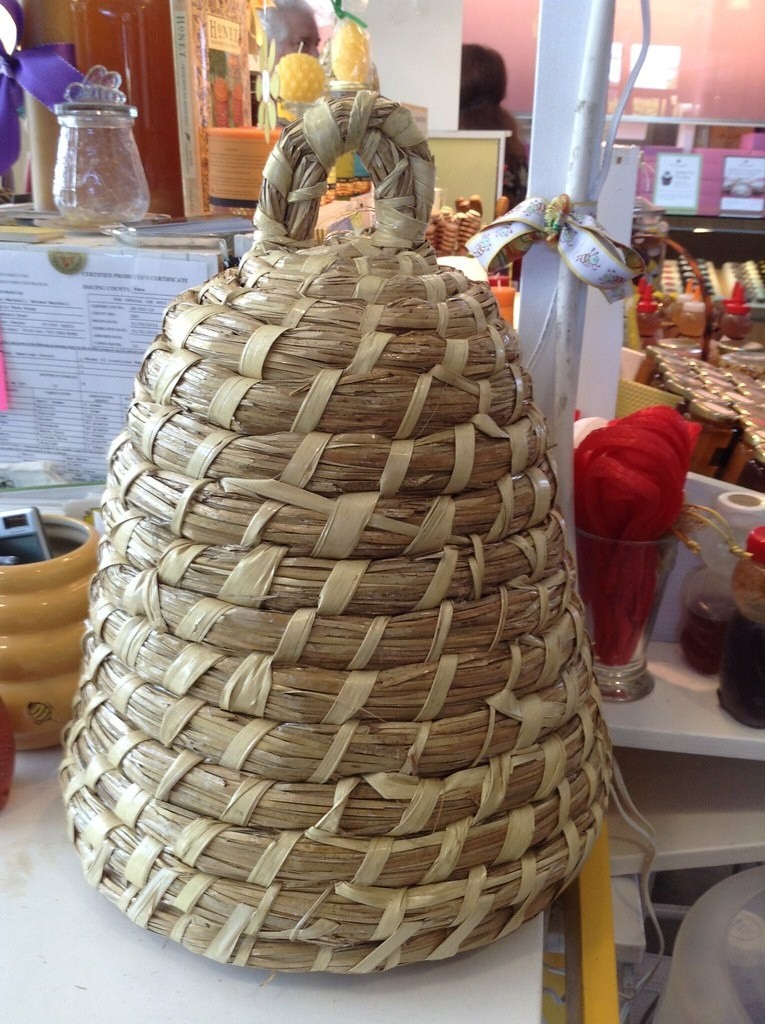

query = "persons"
[458,43,529,211]
[258,0,320,74]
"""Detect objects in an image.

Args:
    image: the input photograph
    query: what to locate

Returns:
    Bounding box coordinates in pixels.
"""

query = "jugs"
[52,65,150,223]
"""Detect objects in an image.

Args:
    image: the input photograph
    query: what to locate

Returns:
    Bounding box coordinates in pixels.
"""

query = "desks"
[558,641,764,1024]
[0,742,545,1024]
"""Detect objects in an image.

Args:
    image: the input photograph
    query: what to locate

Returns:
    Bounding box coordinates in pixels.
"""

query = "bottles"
[682,492,765,673]
[638,277,751,340]
[720,526,765,730]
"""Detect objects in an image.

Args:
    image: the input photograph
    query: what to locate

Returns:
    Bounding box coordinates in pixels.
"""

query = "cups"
[489,277,509,287]
[575,527,679,702]
[491,286,515,327]
[0,515,100,750]
